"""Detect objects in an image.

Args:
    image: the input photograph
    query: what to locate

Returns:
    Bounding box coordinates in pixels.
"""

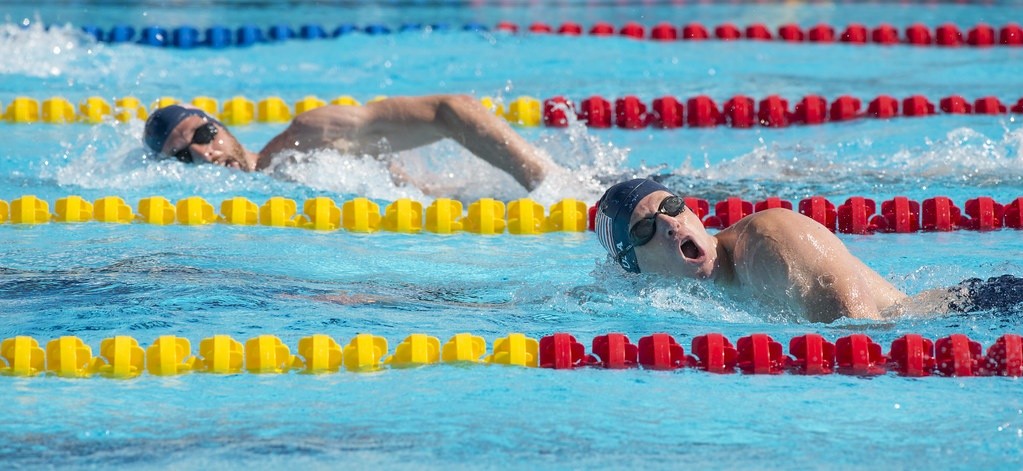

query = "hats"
[595,178,676,274]
[143,104,222,154]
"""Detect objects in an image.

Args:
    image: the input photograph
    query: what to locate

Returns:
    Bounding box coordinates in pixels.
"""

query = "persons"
[142,92,581,194]
[594,177,905,321]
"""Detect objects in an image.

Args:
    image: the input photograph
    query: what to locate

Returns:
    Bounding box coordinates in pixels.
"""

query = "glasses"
[172,121,218,164]
[628,195,685,247]
